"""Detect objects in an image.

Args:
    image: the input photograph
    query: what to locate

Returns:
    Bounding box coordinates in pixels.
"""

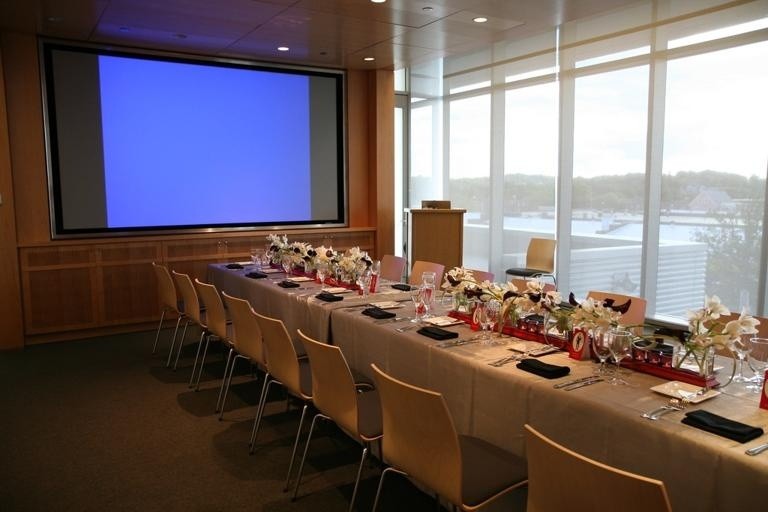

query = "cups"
[441,290,453,309]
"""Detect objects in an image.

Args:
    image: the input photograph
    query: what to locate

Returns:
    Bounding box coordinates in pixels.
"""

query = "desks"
[211,254,768,511]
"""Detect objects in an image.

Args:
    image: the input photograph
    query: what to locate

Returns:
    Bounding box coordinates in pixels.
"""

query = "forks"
[639,397,686,425]
[681,386,711,405]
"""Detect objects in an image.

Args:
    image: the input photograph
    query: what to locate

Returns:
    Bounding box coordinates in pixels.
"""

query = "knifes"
[553,375,604,393]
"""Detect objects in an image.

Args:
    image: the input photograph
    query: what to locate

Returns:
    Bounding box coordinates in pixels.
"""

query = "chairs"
[585,290,648,336]
[409,260,445,290]
[378,254,406,284]
[152,261,209,368]
[464,269,495,286]
[170,268,232,392]
[291,326,383,511]
[368,362,527,511]
[218,290,266,447]
[505,237,557,281]
[519,422,673,511]
[701,308,767,362]
[250,307,375,495]
[510,279,556,293]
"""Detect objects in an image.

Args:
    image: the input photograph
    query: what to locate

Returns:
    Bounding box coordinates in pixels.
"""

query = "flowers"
[266,234,373,283]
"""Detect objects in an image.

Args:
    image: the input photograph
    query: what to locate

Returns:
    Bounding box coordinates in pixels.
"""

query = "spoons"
[525,344,554,355]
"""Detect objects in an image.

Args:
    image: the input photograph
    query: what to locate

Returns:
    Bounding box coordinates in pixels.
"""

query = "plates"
[650,379,721,406]
[508,338,558,358]
[424,317,463,327]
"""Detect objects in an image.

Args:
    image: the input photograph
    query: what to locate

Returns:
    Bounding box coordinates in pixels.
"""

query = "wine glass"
[407,270,437,324]
[723,323,767,393]
[476,299,503,342]
[593,323,632,388]
[249,246,384,302]
